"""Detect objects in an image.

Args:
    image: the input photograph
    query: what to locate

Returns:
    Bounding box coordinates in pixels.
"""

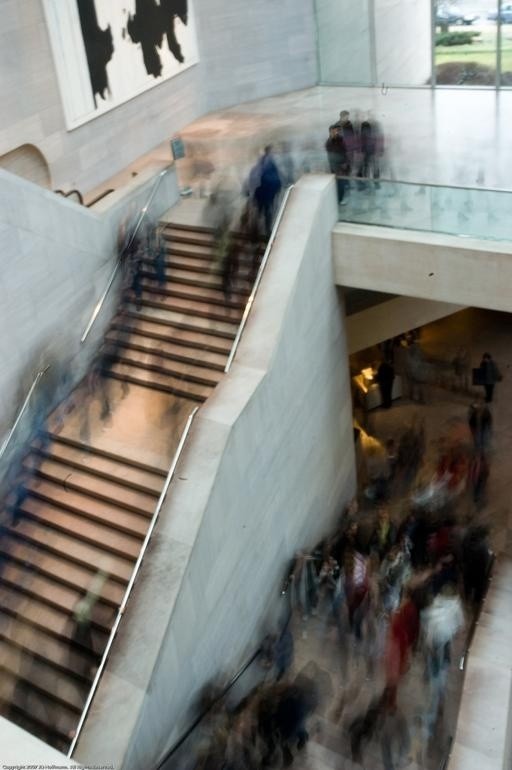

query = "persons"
[0,106,507,770]
